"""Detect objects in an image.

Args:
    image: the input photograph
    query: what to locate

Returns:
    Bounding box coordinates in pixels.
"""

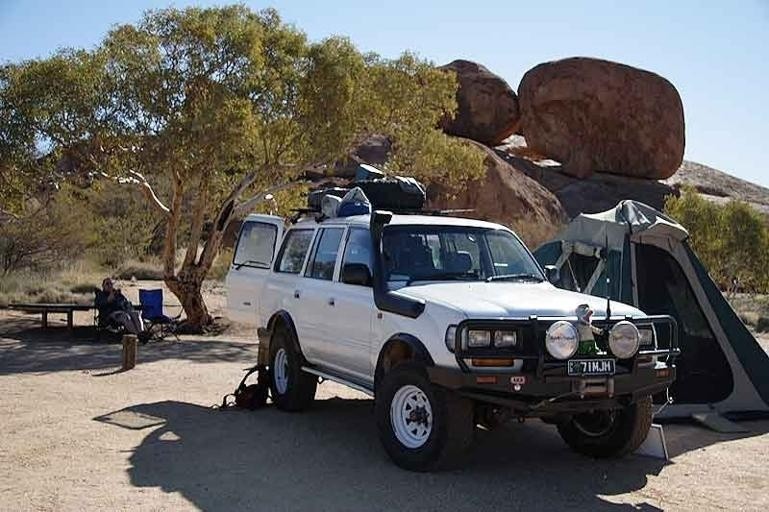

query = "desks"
[9,303,140,341]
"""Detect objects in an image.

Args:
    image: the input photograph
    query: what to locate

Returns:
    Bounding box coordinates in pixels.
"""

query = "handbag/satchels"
[233,384,269,409]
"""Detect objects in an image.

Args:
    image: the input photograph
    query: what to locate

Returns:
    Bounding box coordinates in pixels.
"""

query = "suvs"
[222,196,680,472]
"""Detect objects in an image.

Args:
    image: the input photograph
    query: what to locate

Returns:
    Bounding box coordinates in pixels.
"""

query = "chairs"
[387,248,444,280]
[93,287,121,344]
[139,288,181,341]
[445,251,474,275]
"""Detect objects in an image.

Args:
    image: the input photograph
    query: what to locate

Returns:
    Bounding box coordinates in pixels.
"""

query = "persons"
[95,278,151,344]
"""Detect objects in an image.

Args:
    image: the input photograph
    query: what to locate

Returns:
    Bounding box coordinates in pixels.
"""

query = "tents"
[529,200,769,421]
